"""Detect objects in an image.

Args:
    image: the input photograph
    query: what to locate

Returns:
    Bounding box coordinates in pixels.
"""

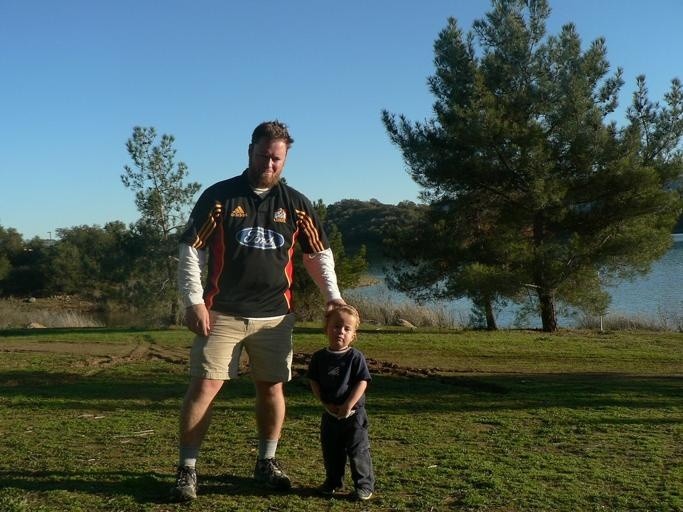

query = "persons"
[308,305,374,501]
[171,119,345,501]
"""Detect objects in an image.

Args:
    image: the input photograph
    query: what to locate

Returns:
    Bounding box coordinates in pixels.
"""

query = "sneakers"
[254,456,291,488]
[176,466,198,499]
[355,488,373,500]
[317,482,344,493]
